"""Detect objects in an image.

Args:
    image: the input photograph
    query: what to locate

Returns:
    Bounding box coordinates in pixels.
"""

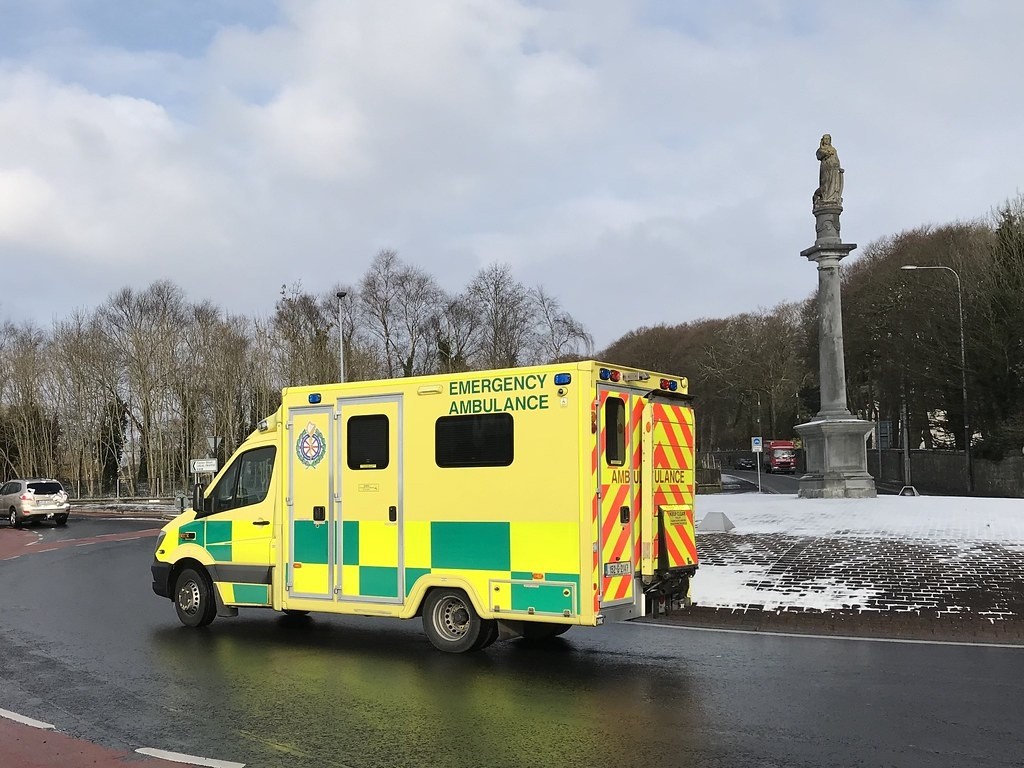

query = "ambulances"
[150,359,698,653]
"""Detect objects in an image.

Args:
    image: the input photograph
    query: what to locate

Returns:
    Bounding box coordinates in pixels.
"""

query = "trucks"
[763,440,797,474]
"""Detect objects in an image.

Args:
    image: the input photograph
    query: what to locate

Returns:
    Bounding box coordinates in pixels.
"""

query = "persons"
[815,134,845,203]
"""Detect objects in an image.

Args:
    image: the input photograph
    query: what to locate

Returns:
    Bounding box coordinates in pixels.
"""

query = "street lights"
[782,378,805,473]
[746,388,762,437]
[901,265,975,496]
[336,291,346,383]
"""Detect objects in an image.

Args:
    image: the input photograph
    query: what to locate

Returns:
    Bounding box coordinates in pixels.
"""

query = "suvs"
[0,476,70,528]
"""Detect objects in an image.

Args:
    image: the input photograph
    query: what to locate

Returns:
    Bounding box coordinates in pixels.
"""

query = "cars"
[734,458,755,470]
